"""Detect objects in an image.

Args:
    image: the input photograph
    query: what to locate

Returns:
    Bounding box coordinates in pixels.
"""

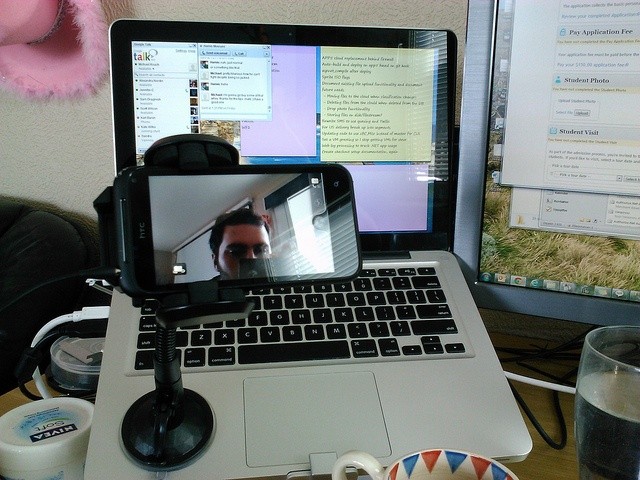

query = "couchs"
[0,194,113,395]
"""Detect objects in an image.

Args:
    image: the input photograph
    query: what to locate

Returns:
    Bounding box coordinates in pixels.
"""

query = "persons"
[208,208,274,281]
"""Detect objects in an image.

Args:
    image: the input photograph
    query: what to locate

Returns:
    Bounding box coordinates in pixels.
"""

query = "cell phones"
[115,164,363,296]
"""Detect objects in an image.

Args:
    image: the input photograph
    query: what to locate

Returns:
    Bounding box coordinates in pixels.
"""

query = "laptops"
[84,19,533,479]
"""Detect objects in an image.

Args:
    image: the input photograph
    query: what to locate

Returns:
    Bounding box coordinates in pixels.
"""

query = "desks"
[1,306,639,479]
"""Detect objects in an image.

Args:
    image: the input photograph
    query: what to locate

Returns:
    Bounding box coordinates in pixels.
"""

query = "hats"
[0,0,108,98]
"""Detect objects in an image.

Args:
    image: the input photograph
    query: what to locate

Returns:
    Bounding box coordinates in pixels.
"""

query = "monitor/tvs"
[454,0,640,330]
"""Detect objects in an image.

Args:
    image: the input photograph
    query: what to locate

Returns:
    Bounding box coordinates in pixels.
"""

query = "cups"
[331,448,519,480]
[574,325,640,479]
